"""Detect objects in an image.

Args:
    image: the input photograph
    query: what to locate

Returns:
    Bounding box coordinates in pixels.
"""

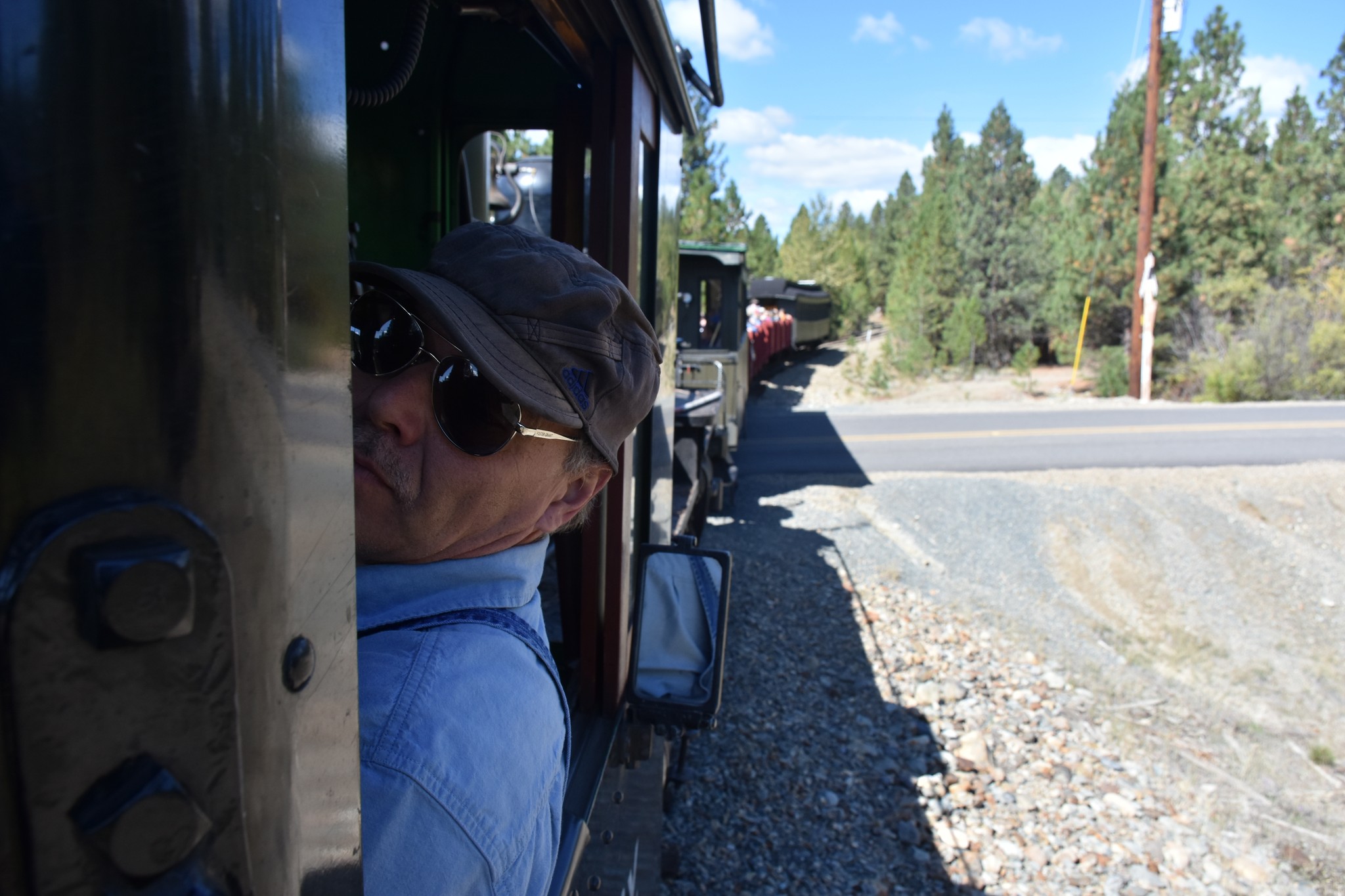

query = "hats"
[350,220,663,474]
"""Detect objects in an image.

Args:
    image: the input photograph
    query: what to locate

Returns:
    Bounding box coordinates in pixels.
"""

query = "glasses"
[347,287,585,458]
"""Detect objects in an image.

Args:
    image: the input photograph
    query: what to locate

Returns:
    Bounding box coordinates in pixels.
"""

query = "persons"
[349,223,664,896]
[634,551,723,707]
[746,299,792,333]
[869,304,887,330]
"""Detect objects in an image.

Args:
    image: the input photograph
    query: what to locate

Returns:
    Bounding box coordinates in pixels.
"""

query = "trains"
[3,0,832,896]
